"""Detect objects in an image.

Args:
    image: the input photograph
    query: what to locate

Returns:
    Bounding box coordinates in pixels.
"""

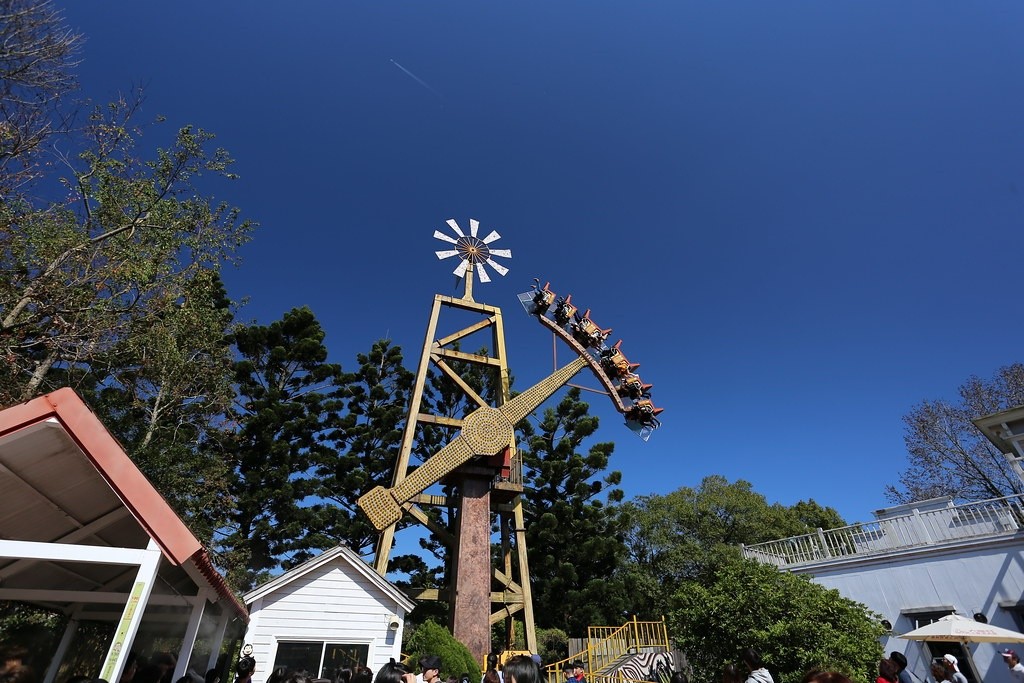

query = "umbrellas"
[894,613,1024,683]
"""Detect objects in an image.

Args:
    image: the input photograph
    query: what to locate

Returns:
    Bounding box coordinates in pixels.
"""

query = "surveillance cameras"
[390,614,400,630]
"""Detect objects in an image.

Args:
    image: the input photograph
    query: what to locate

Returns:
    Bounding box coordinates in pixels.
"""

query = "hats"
[418,656,441,669]
[944,654,960,672]
[531,655,542,666]
[998,648,1015,657]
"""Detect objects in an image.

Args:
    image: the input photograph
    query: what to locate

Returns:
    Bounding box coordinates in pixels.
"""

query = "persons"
[889,651,913,683]
[562,663,577,683]
[941,653,969,683]
[930,663,952,683]
[800,669,851,683]
[876,659,899,683]
[530,277,662,430]
[1000,647,1024,683]
[743,648,775,683]
[0,645,549,683]
[572,660,588,683]
